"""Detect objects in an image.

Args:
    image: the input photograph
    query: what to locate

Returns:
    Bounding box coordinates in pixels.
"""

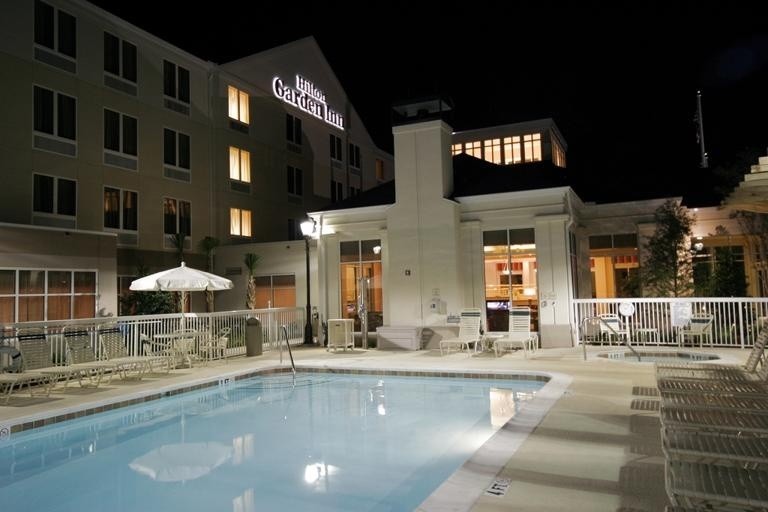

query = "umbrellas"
[129,262,233,364]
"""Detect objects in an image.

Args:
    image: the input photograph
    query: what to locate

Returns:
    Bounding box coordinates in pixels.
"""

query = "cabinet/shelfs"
[327,318,355,353]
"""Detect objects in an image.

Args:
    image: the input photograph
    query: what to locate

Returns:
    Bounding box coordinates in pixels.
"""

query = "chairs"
[652,313,767,511]
[439,307,489,356]
[596,314,629,346]
[494,305,531,358]
[0,323,233,406]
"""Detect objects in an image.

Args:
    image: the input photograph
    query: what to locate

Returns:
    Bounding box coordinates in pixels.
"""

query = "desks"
[486,329,539,351]
[424,322,461,338]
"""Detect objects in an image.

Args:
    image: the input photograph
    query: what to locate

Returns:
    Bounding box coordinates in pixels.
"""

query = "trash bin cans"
[245,317,262,356]
